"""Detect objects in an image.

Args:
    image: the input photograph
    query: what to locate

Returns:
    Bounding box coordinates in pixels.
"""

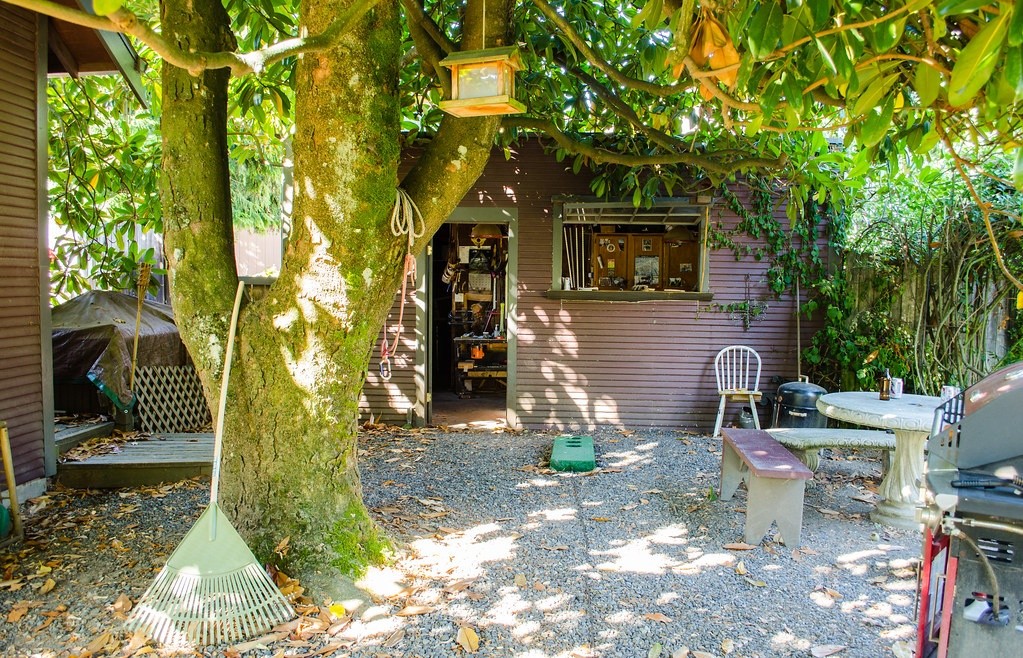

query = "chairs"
[714,344,763,438]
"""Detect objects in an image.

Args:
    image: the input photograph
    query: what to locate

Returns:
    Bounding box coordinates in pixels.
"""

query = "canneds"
[891,378,903,399]
[941,385,960,404]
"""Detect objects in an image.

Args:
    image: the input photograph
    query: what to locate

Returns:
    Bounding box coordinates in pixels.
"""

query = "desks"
[453,332,508,399]
[816,392,965,531]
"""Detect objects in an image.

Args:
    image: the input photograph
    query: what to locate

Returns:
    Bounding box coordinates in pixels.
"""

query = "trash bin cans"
[773,375,828,428]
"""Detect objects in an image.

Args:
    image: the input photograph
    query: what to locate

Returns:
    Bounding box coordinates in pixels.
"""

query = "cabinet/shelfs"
[592,232,668,291]
[442,245,502,334]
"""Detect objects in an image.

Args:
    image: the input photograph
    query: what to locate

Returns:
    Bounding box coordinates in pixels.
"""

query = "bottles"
[880,368,891,400]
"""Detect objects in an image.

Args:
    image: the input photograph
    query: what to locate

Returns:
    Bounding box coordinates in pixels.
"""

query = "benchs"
[720,426,814,549]
[763,428,896,473]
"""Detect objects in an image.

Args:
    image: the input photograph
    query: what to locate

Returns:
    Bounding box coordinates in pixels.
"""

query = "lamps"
[437,0,528,118]
[469,224,504,238]
